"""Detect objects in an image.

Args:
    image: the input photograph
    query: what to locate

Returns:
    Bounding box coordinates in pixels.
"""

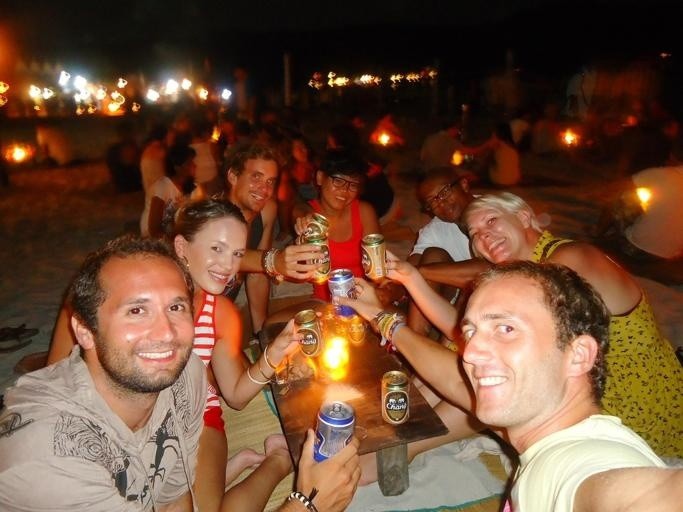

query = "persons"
[383,192,682,468]
[0,231,361,511]
[1,95,683,228]
[140,146,202,243]
[292,152,382,302]
[177,141,325,334]
[106,121,139,193]
[589,146,683,259]
[376,165,480,338]
[43,195,305,512]
[330,261,682,511]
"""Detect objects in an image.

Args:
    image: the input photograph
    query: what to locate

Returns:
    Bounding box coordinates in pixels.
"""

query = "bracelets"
[284,487,321,512]
[256,357,271,380]
[258,245,284,285]
[369,308,408,350]
[263,343,278,370]
[246,364,271,385]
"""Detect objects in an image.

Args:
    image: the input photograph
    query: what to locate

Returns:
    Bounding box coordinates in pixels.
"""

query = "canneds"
[294,212,330,245]
[325,311,367,347]
[381,371,411,425]
[327,268,357,318]
[313,401,355,463]
[294,309,322,358]
[361,233,386,280]
[306,235,331,285]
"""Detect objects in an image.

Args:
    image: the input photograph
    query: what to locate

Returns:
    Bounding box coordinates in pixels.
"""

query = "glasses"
[324,173,362,192]
[424,180,459,212]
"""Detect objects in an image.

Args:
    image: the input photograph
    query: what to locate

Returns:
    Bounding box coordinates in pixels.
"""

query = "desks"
[257,321,449,496]
